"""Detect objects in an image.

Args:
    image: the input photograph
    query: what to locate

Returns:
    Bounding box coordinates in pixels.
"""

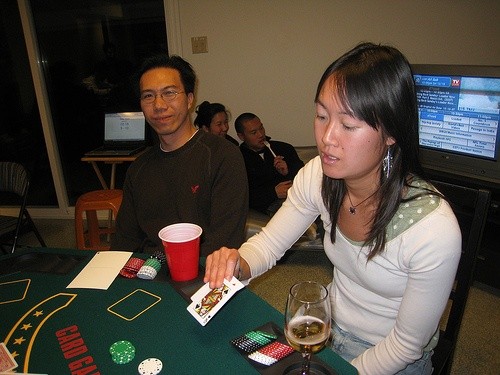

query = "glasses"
[141,90,185,103]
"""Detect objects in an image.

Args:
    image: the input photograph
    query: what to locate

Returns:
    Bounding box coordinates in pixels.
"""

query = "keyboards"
[84,150,137,157]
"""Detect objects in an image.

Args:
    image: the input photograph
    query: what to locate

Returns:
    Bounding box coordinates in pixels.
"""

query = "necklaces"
[346,187,379,214]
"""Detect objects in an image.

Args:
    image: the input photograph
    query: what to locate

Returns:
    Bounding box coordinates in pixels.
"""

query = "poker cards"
[0,342,19,374]
[187,275,245,327]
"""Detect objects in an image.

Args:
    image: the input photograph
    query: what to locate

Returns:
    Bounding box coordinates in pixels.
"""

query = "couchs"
[245,144,323,252]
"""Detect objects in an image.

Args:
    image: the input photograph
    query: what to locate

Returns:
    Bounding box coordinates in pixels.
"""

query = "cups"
[158,223,202,282]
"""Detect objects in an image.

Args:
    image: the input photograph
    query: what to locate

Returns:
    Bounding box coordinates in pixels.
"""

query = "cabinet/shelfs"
[425,168,500,290]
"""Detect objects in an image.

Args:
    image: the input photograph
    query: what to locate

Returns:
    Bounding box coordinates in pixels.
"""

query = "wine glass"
[284,281,331,375]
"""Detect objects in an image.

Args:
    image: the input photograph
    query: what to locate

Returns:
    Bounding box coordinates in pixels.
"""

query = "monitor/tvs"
[409,62,500,190]
[103,110,148,148]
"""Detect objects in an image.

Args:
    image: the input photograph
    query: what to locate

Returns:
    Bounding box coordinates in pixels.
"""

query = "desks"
[0,245,360,375]
[81,141,153,242]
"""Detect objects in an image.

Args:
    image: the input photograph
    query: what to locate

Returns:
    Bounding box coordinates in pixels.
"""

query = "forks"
[264,141,276,158]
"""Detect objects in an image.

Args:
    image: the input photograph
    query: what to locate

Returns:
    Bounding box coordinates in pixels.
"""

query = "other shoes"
[306,222,318,240]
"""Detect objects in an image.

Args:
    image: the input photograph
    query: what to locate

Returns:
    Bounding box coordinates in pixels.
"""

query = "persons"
[108,56,250,257]
[203,42,463,374]
[234,112,325,245]
[193,101,240,147]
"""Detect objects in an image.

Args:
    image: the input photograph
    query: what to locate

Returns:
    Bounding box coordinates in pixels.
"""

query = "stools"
[75,189,124,251]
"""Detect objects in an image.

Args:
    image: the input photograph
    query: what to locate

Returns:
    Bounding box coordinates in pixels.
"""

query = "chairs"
[425,181,489,375]
[0,162,48,253]
